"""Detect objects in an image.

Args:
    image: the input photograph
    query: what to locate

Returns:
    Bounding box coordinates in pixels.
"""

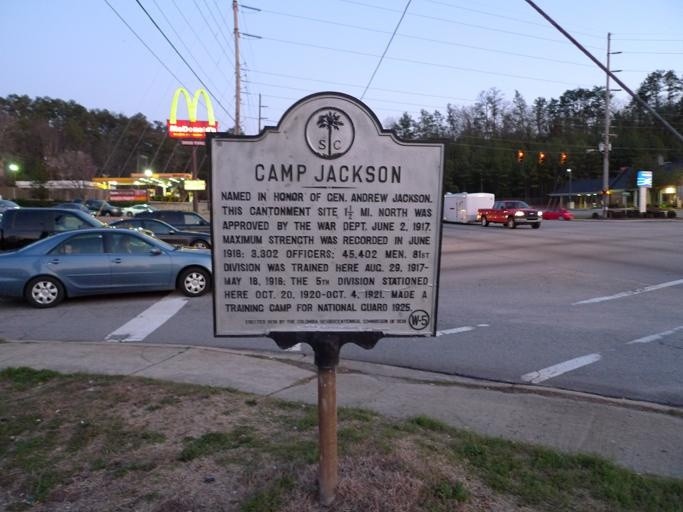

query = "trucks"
[443,193,494,225]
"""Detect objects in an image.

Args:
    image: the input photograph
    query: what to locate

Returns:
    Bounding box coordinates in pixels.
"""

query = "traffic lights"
[538,153,545,165]
[560,154,566,165]
[518,151,524,164]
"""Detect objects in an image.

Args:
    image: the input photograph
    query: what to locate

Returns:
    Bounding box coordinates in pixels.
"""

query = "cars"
[0,227,213,308]
[122,204,153,218]
[136,211,211,232]
[543,208,573,221]
[110,217,212,249]
[0,199,119,245]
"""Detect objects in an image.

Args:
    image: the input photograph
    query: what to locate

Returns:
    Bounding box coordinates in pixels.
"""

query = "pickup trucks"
[478,200,543,229]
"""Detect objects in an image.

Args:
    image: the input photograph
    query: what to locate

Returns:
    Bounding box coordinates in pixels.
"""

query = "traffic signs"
[636,171,653,187]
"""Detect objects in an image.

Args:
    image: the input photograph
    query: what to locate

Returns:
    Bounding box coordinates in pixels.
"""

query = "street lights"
[144,169,153,203]
[8,164,21,197]
